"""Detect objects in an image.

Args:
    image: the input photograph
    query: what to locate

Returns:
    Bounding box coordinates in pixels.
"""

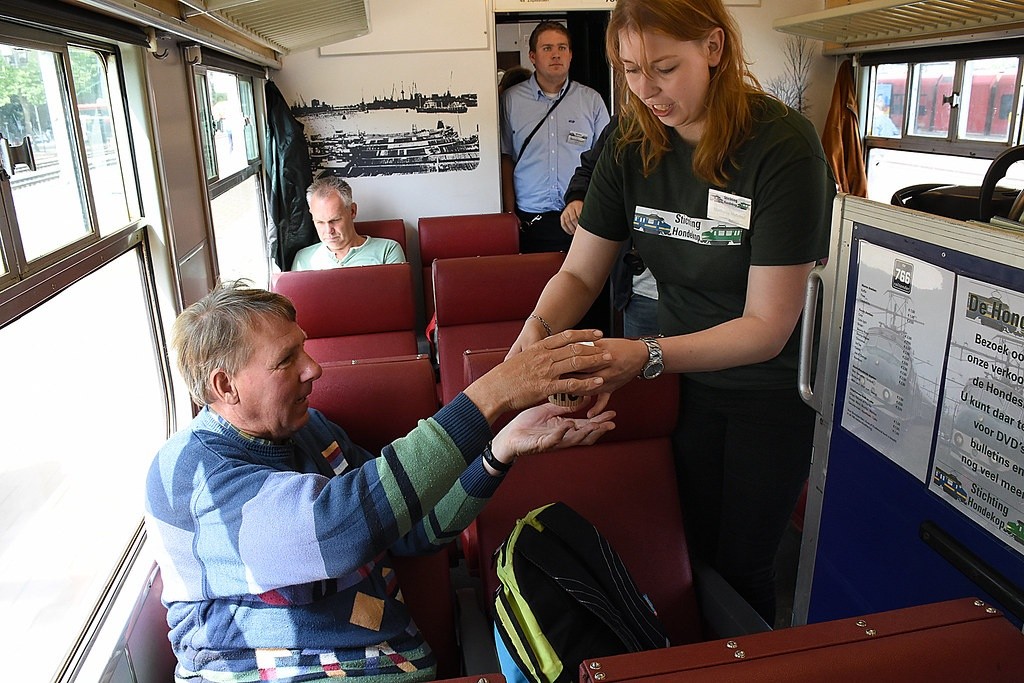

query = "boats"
[288,70,478,119]
[305,122,480,180]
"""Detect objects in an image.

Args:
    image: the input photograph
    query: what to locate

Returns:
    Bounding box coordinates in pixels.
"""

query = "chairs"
[463,334,702,645]
[354,219,406,253]
[419,212,519,359]
[431,252,565,408]
[306,353,467,678]
[269,262,417,362]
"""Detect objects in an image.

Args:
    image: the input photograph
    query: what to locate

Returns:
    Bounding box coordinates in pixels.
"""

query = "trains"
[876,73,1015,136]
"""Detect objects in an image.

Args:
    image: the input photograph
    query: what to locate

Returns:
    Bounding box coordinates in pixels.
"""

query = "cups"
[544,335,594,407]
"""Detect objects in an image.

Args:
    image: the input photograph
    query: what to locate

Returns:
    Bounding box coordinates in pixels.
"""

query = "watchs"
[636,338,665,381]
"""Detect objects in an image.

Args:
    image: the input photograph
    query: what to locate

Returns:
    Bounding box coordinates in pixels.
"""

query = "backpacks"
[491,501,671,683]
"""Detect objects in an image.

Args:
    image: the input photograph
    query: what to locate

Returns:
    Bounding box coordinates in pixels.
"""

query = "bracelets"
[482,438,519,471]
[526,315,552,337]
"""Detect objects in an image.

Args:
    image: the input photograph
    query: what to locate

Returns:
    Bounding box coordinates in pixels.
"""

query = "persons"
[290,177,407,273]
[144,277,616,683]
[503,0,840,637]
[495,22,661,342]
